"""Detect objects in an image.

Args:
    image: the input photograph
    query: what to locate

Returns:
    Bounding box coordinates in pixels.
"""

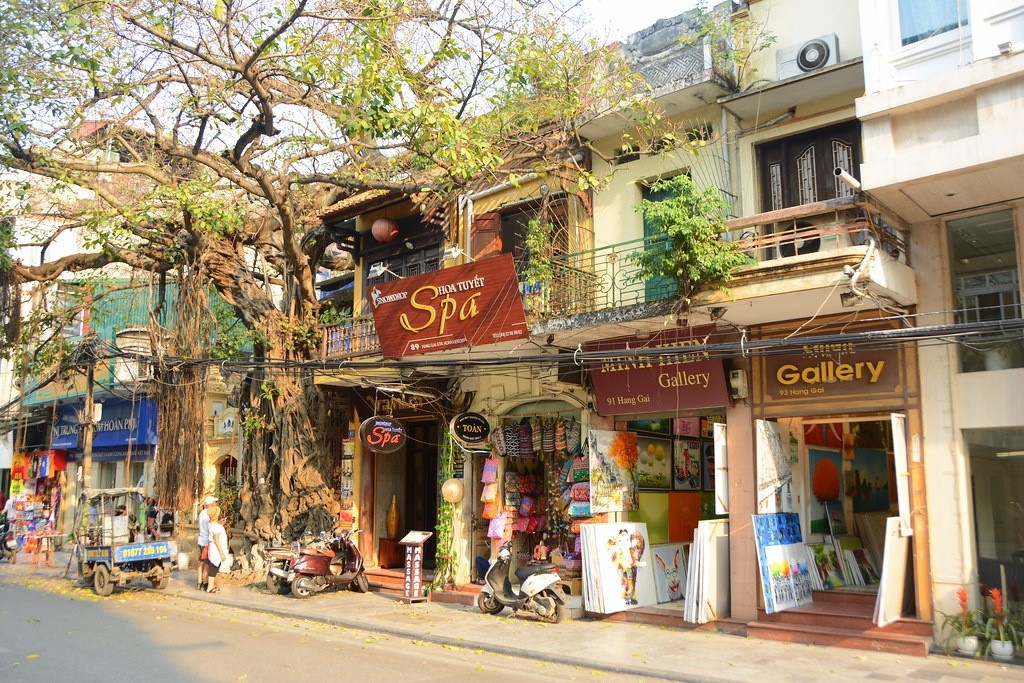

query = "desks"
[21,534,69,570]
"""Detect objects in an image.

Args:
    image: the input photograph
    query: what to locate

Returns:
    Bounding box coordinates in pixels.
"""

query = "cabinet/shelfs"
[379,537,405,569]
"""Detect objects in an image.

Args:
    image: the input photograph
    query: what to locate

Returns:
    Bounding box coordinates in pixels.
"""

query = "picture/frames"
[805,444,890,545]
[625,414,725,491]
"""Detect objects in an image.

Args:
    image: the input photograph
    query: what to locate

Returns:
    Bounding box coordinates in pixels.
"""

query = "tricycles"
[77,487,177,597]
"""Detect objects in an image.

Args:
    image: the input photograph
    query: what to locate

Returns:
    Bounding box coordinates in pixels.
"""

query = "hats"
[207,505,221,522]
[202,495,221,505]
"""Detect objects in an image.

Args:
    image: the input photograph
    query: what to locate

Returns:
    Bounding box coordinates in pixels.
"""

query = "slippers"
[216,587,221,593]
[207,588,218,594]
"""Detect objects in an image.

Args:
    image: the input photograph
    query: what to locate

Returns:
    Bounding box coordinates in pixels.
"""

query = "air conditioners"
[776,211,852,258]
[776,33,840,81]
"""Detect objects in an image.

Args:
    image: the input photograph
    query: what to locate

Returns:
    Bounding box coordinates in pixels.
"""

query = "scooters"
[257,516,371,599]
[476,537,579,624]
[0,525,20,564]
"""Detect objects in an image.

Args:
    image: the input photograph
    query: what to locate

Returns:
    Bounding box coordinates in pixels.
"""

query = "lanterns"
[371,217,399,243]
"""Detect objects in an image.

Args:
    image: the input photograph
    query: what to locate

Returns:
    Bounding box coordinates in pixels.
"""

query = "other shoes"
[195,584,204,589]
[206,583,207,591]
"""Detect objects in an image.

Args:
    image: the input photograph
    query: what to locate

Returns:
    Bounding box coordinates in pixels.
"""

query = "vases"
[955,635,979,651]
[990,639,1014,656]
[387,494,400,538]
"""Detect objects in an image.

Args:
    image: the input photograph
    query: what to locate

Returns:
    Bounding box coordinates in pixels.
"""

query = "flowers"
[933,587,1024,662]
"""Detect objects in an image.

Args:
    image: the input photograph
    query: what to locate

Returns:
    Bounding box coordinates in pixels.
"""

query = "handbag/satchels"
[201,546,210,561]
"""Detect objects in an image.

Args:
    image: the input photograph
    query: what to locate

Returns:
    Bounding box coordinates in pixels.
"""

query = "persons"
[140,497,160,541]
[115,506,138,542]
[196,497,219,591]
[207,506,228,593]
[0,491,17,530]
